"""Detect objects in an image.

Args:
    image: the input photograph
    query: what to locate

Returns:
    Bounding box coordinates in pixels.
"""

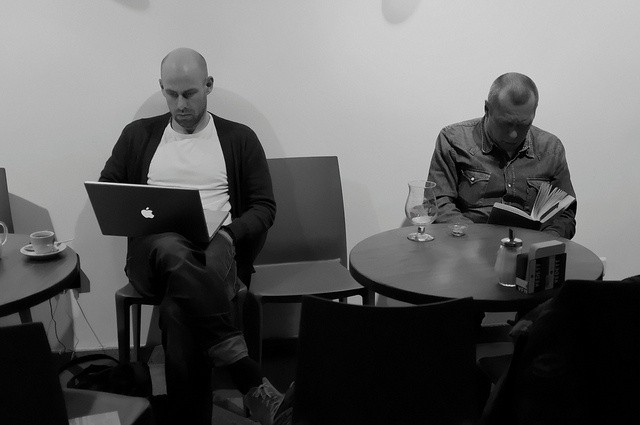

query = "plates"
[20,241,68,261]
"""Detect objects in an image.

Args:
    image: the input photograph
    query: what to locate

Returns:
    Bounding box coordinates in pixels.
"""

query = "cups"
[448,223,465,237]
[30,231,56,252]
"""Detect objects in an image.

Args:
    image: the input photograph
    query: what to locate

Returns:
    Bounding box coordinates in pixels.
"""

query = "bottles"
[497,236,523,288]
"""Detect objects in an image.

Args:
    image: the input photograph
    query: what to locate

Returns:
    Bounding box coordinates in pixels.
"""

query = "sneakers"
[242,367,296,424]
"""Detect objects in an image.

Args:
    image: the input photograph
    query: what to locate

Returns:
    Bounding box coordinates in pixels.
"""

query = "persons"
[84,48,297,425]
[423,72,577,240]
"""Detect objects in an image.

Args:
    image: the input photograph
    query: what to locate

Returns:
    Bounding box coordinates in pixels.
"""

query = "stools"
[112,250,213,379]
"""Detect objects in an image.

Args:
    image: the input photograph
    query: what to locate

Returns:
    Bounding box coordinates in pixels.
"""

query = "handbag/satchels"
[67,354,152,405]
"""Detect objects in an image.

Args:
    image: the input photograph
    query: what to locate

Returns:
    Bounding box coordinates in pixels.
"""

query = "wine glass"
[404,180,442,242]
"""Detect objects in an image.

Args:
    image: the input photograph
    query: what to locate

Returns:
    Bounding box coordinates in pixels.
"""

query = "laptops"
[84,181,231,245]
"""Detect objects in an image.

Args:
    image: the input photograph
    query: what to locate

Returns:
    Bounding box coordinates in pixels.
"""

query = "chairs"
[0,324,150,425]
[241,155,364,379]
[479,274,639,425]
[0,169,32,323]
[296,295,479,424]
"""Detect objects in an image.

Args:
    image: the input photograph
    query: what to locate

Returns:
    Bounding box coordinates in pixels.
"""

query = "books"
[488,181,575,231]
